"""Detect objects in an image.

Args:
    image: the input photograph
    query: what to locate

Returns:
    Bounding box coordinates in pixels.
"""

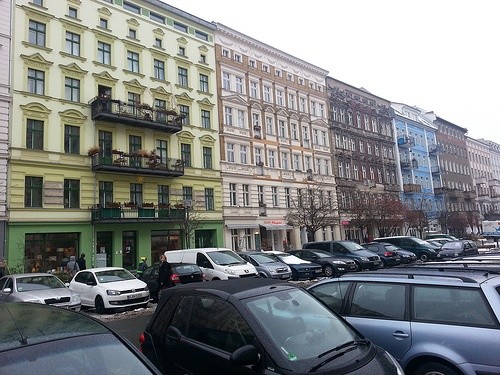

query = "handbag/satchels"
[73,262,77,276]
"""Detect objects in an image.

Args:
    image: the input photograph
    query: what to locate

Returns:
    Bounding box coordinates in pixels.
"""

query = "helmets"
[141,257,147,261]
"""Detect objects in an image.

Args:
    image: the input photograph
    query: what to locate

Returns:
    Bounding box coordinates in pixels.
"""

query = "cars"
[359,234,500,276]
[257,264,500,375]
[283,249,357,278]
[139,278,407,375]
[68,267,150,314]
[138,261,205,303]
[0,272,82,312]
[234,250,294,281]
[0,301,164,375]
[263,250,323,281]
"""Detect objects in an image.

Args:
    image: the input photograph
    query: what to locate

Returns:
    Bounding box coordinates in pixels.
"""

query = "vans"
[164,248,261,281]
[302,240,382,272]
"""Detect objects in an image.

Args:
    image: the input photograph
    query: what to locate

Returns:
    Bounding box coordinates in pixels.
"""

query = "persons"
[365,232,372,241]
[137,255,174,304]
[27,246,86,276]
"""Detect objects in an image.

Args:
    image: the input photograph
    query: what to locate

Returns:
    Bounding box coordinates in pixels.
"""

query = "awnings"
[259,223,297,230]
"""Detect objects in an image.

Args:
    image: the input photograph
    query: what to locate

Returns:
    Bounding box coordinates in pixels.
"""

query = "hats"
[70,256,75,260]
